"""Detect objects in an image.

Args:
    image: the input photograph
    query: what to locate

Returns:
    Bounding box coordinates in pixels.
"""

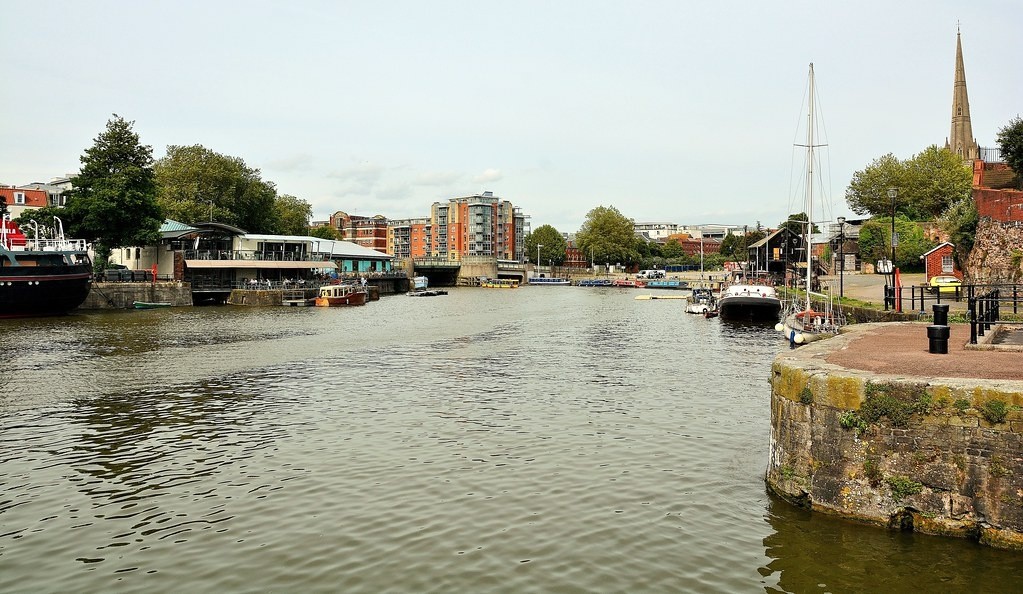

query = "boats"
[781,297,846,345]
[686,286,718,315]
[316,284,369,307]
[407,269,732,299]
[0,211,95,315]
[719,275,783,327]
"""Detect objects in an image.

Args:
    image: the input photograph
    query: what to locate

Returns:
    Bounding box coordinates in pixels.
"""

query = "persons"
[185,249,408,289]
[625,276,628,280]
[721,273,773,298]
[640,270,665,279]
